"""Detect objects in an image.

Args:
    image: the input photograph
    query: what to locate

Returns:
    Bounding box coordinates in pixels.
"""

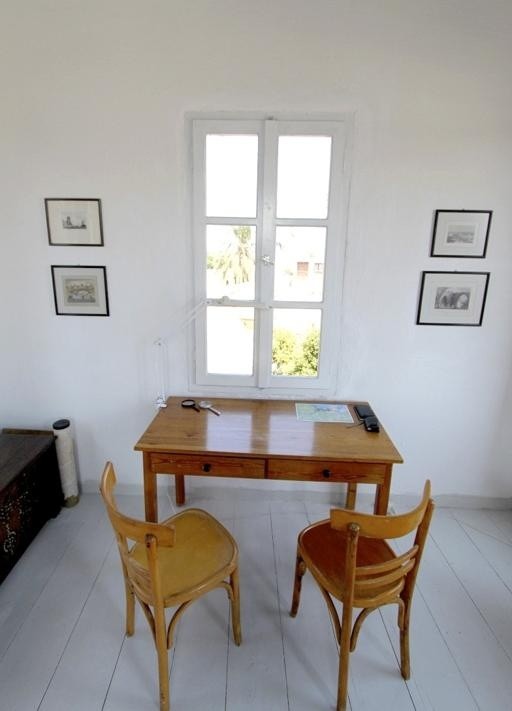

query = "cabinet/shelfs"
[0,428,65,587]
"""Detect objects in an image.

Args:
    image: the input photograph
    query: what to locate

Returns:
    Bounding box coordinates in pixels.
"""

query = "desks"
[134,396,404,524]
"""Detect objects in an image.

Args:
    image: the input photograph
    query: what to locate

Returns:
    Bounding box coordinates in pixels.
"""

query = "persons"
[81,221,86,228]
[67,216,71,225]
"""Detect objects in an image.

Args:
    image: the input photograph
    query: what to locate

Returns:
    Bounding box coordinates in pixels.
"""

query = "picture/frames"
[44,198,104,249]
[430,209,493,259]
[51,265,110,316]
[416,271,491,328]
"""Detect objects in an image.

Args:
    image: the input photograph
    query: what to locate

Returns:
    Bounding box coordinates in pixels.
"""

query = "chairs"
[290,479,436,711]
[99,461,244,711]
